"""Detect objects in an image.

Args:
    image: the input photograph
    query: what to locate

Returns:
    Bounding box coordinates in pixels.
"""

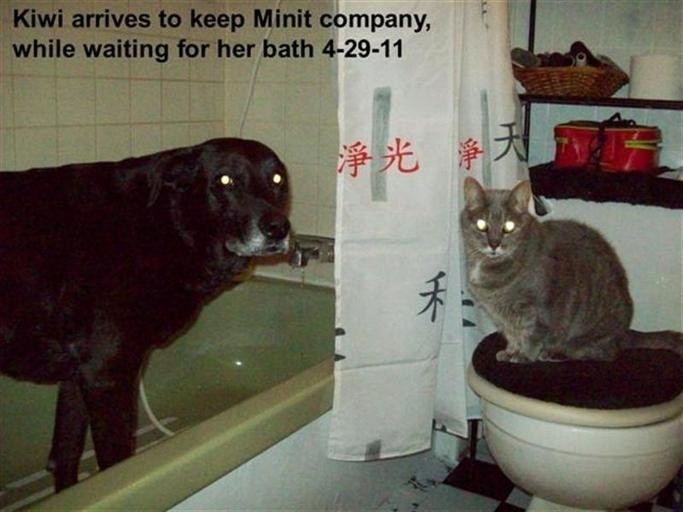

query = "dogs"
[1,136,293,493]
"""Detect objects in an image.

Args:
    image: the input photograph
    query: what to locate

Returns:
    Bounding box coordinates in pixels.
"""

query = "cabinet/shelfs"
[506,95,683,210]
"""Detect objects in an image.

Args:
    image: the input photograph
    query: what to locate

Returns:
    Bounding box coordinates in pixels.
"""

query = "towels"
[628,55,683,102]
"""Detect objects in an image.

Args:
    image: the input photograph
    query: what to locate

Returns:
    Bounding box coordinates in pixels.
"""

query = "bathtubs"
[1,273,415,512]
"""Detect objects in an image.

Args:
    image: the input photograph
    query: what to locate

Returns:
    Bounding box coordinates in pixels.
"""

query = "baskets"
[509,66,631,98]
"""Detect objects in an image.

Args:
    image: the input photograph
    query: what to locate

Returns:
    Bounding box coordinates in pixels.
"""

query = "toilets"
[466,161,683,512]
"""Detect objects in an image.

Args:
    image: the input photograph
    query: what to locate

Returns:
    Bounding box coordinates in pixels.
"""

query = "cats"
[458,173,683,366]
